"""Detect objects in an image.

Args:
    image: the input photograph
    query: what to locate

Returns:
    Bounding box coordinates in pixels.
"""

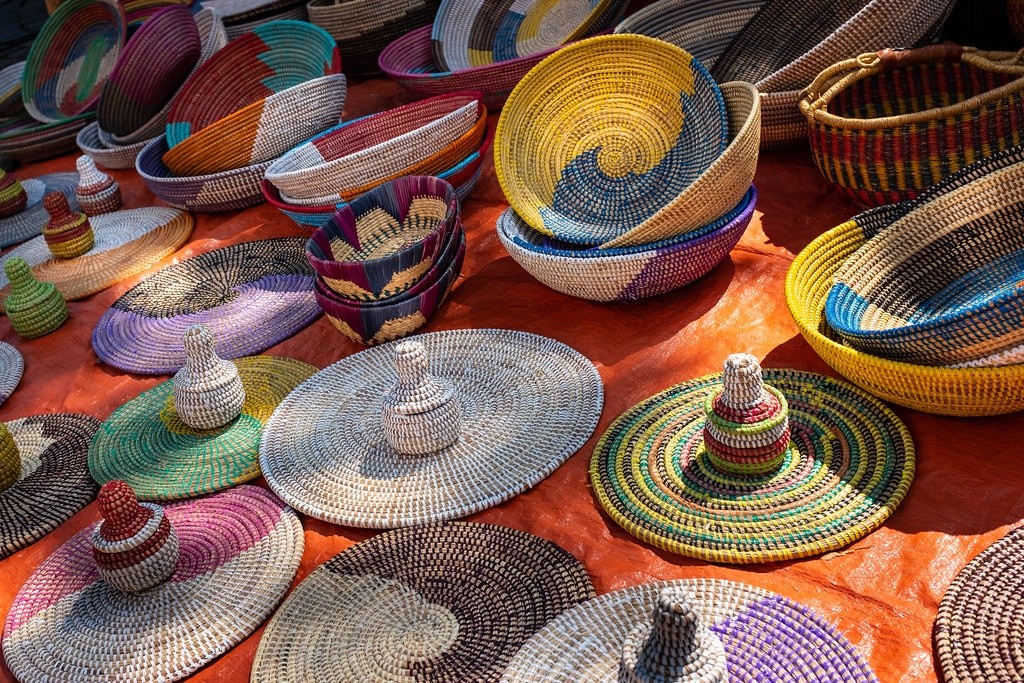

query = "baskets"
[0,0,1024,415]
